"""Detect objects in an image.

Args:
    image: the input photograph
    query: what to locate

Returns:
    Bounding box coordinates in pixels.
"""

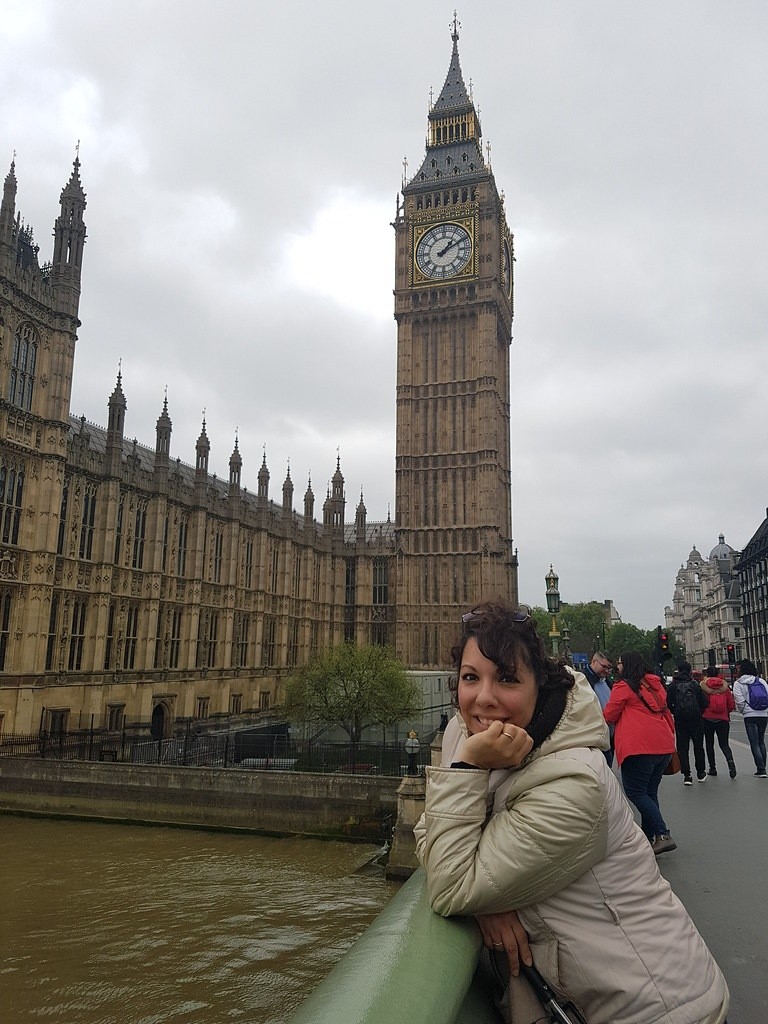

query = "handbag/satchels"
[664,751,681,775]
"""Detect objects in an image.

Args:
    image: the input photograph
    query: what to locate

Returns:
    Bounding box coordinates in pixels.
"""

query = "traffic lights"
[727,644,735,662]
[661,634,668,651]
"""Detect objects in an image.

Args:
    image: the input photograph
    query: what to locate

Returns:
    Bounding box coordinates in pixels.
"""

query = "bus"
[690,671,702,682]
[702,664,741,688]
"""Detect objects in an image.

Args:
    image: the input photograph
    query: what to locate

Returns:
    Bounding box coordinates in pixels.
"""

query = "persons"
[732,659,768,778]
[573,650,615,770]
[414,599,730,1024]
[698,667,736,779]
[666,661,709,786]
[602,650,677,855]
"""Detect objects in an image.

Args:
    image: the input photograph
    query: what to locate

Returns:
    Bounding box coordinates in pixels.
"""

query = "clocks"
[417,224,472,279]
[502,236,512,301]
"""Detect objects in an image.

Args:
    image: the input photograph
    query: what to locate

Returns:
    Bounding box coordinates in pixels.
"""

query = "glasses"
[461,610,539,642]
[597,657,613,672]
[616,661,623,666]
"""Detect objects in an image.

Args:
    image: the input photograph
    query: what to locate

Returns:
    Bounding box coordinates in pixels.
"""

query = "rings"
[502,732,513,739]
[493,942,503,946]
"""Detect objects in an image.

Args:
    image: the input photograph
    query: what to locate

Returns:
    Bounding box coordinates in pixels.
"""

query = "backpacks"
[674,680,702,720]
[745,677,768,710]
[709,694,725,713]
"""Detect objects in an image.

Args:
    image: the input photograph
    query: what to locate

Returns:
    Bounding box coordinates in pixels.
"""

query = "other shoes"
[697,771,707,782]
[708,769,716,775]
[728,760,737,779]
[653,832,677,855]
[752,770,767,777]
[684,776,692,785]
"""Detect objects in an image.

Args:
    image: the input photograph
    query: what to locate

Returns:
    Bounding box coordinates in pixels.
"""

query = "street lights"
[545,564,561,660]
[596,633,600,650]
[563,626,570,648]
